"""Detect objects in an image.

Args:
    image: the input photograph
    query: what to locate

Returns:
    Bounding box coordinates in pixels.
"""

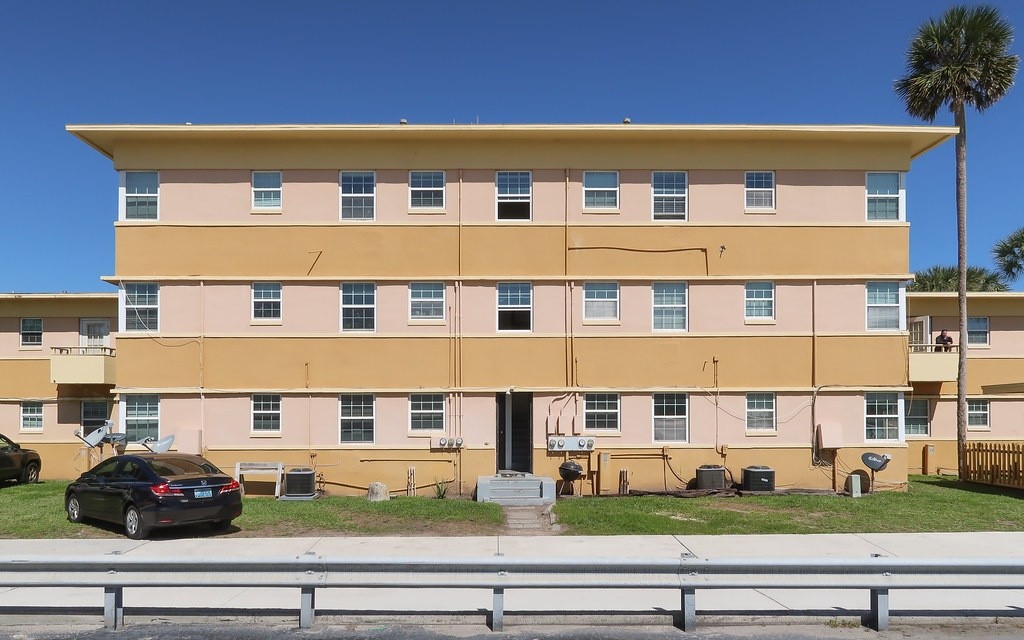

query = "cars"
[0,432,42,488]
[63,451,244,541]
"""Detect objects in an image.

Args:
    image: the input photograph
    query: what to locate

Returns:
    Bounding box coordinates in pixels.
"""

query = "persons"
[935,330,953,352]
